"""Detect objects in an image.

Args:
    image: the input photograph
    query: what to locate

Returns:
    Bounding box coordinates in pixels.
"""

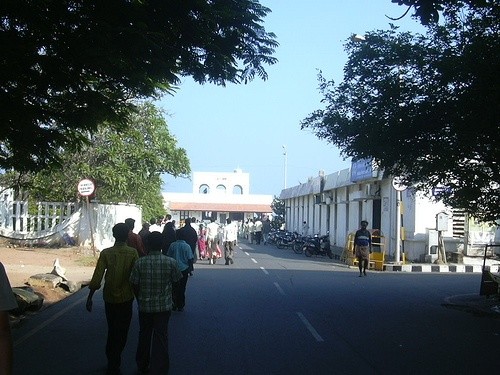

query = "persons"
[353,220,372,277]
[0,261,17,375]
[86,215,310,375]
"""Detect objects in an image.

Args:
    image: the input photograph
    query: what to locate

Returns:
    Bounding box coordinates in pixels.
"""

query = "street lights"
[282,144,288,190]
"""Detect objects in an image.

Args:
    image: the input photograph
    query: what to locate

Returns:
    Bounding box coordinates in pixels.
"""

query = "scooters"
[263,229,331,258]
